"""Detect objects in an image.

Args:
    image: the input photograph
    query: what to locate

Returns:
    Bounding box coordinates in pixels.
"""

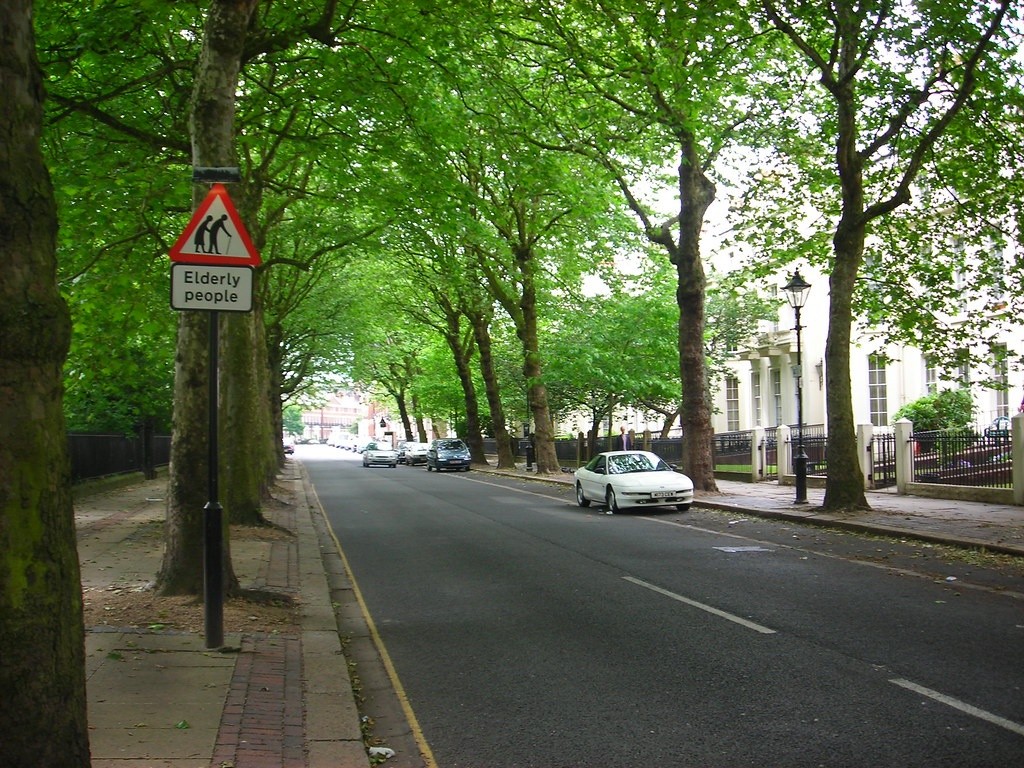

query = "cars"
[574,450,694,514]
[362,441,398,468]
[283,442,295,456]
[294,437,327,444]
[396,441,430,465]
[327,432,375,453]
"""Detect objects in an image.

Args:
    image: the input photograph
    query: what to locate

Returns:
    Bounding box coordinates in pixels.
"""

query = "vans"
[427,438,472,472]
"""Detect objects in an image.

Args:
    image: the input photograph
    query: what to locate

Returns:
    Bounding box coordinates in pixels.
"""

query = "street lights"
[778,266,811,504]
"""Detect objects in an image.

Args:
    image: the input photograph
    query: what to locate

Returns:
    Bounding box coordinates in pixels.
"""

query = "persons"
[616,426,631,451]
